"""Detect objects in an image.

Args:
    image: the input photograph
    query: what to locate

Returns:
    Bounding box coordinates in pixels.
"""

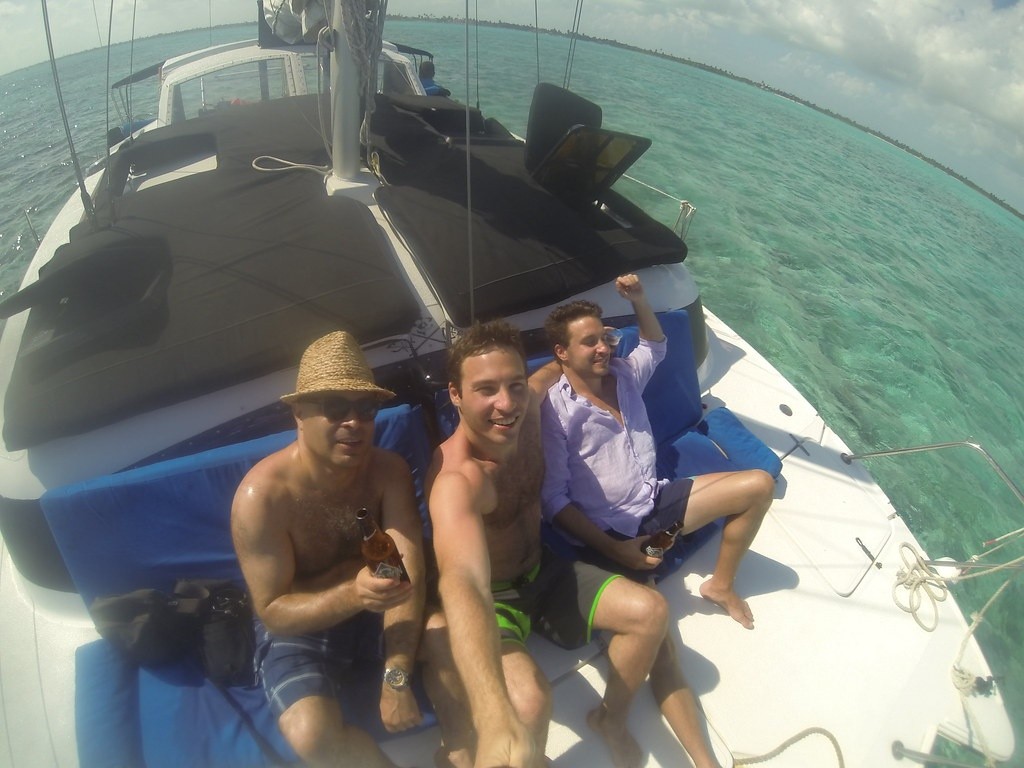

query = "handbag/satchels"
[175,579,253,684]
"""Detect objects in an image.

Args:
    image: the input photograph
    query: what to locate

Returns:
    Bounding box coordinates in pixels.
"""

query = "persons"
[422,318,669,768]
[229,330,477,768]
[535,271,775,768]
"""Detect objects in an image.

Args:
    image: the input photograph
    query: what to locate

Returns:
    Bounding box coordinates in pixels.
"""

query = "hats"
[279,331,396,404]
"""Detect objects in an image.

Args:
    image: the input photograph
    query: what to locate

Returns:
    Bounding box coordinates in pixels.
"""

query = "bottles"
[354,507,412,586]
[642,521,684,560]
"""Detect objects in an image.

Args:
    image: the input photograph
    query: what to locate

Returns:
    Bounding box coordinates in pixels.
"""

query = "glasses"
[301,396,378,422]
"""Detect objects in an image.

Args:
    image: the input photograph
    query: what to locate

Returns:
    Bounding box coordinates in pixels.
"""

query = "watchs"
[375,667,414,691]
